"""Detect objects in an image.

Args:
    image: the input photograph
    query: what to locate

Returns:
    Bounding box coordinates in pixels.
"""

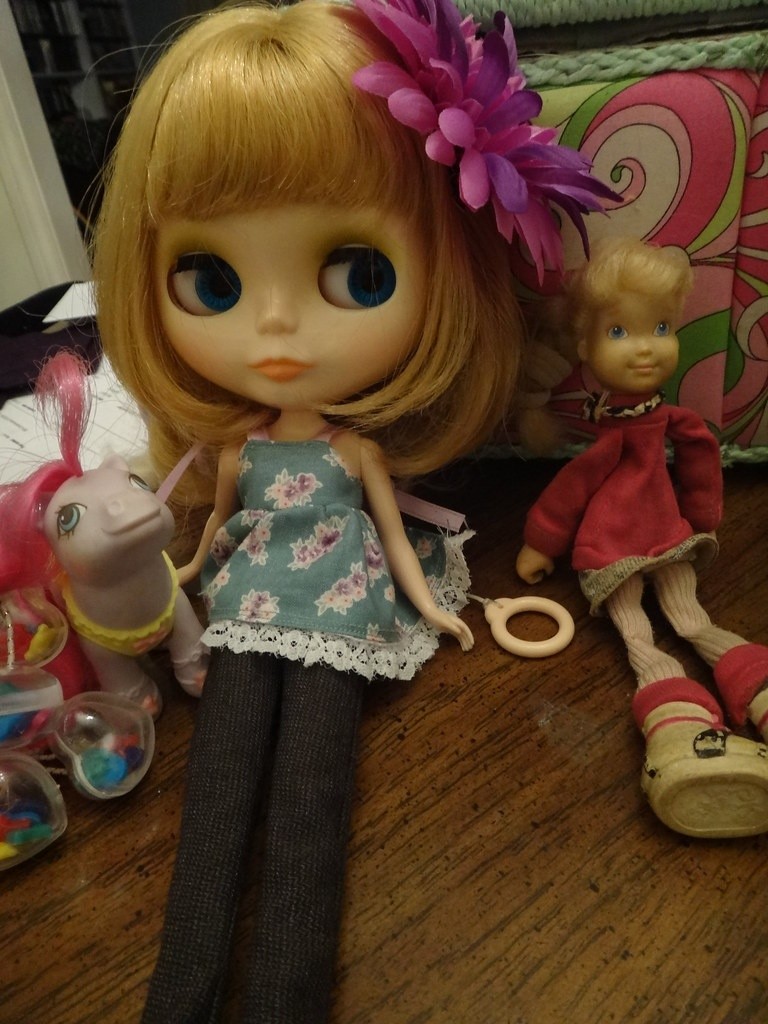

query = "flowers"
[355,0,624,289]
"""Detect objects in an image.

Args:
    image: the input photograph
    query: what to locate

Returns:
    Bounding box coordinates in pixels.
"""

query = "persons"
[90,0,508,1024]
[518,254,765,828]
